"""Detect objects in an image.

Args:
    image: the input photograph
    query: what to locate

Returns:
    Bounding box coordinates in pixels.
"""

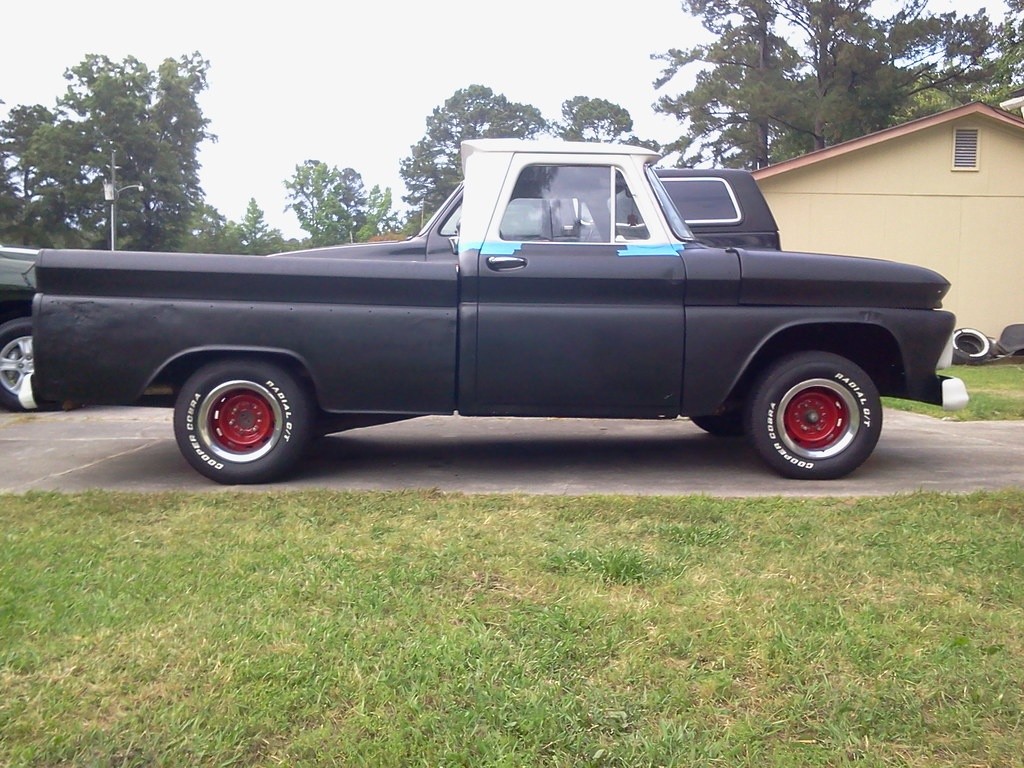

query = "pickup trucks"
[31,135,971,488]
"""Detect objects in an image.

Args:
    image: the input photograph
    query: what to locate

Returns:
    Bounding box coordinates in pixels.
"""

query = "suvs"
[264,161,787,260]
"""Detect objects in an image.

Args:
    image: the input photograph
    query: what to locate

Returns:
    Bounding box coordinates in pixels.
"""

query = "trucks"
[0,243,46,414]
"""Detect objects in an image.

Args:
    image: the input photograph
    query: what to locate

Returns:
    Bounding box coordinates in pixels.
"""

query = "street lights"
[109,184,145,251]
